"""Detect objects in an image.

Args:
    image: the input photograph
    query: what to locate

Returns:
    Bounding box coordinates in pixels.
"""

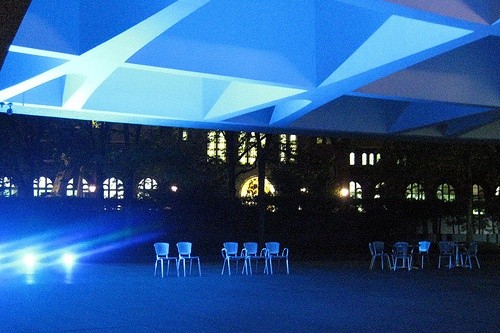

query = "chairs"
[153,243,201,279]
[220,242,289,275]
[368,241,430,273]
[438,241,481,269]
